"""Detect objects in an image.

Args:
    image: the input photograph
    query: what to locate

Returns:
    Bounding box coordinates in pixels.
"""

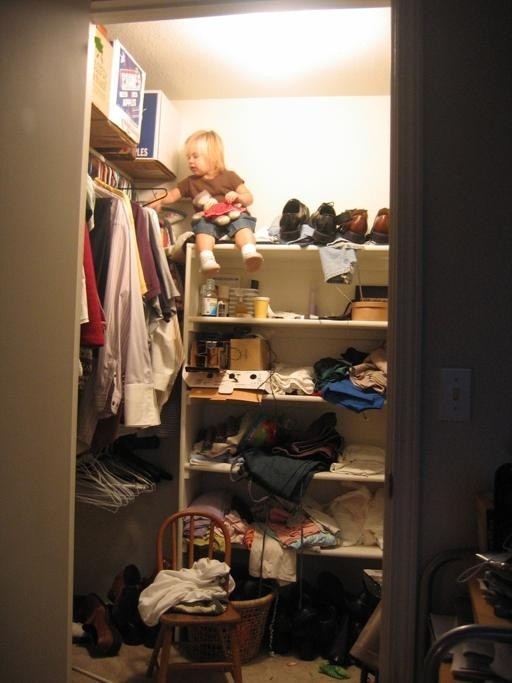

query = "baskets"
[180,576,275,663]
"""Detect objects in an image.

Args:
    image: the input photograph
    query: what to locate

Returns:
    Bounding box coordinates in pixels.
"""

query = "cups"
[253,296,270,319]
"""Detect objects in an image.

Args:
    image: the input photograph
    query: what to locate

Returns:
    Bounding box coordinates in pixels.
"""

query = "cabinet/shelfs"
[424,553,512,683]
[174,240,394,660]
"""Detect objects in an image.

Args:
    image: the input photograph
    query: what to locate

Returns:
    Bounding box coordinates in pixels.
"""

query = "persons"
[147,128,264,276]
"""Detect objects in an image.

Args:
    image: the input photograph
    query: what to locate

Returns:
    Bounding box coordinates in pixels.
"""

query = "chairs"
[145,508,245,682]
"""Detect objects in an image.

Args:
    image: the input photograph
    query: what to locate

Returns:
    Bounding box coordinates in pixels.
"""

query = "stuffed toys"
[190,188,249,227]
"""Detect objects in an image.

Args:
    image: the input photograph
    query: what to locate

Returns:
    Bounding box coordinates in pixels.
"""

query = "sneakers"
[201,261,221,278]
[243,253,263,271]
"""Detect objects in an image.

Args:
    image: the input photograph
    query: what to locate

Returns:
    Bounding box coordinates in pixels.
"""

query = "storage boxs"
[88,20,181,180]
[230,336,270,369]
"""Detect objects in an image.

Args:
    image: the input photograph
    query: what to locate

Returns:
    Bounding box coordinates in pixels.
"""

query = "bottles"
[201,278,218,315]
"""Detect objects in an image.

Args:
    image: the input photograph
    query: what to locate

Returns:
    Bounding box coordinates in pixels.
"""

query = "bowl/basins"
[350,300,387,321]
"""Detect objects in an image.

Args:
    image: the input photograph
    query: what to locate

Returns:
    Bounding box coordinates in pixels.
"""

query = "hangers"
[87,146,169,211]
[76,438,172,512]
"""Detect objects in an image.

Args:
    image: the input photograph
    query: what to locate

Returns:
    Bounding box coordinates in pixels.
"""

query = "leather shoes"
[334,209,368,243]
[372,206,390,244]
[309,201,336,244]
[266,572,378,666]
[278,196,308,240]
[82,565,167,657]
